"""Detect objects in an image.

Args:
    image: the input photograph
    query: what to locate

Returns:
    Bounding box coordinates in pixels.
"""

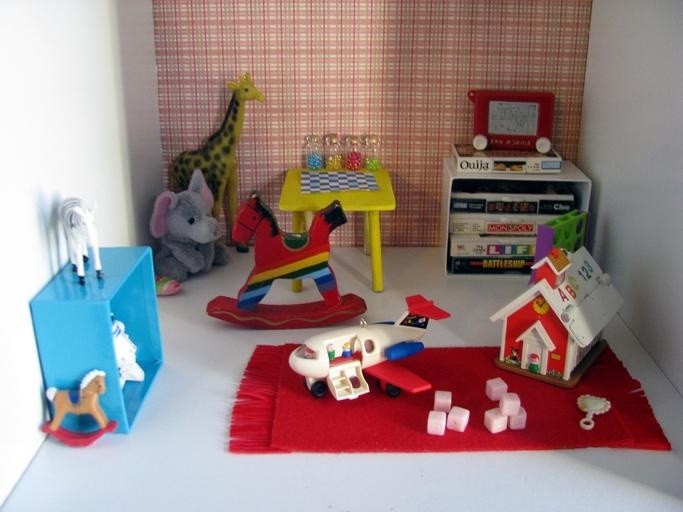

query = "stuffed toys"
[149,167,231,281]
[172,71,265,254]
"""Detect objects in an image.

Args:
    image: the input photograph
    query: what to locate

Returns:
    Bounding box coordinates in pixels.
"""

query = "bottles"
[362,135,380,170]
[344,136,362,171]
[304,135,323,168]
[324,133,344,170]
